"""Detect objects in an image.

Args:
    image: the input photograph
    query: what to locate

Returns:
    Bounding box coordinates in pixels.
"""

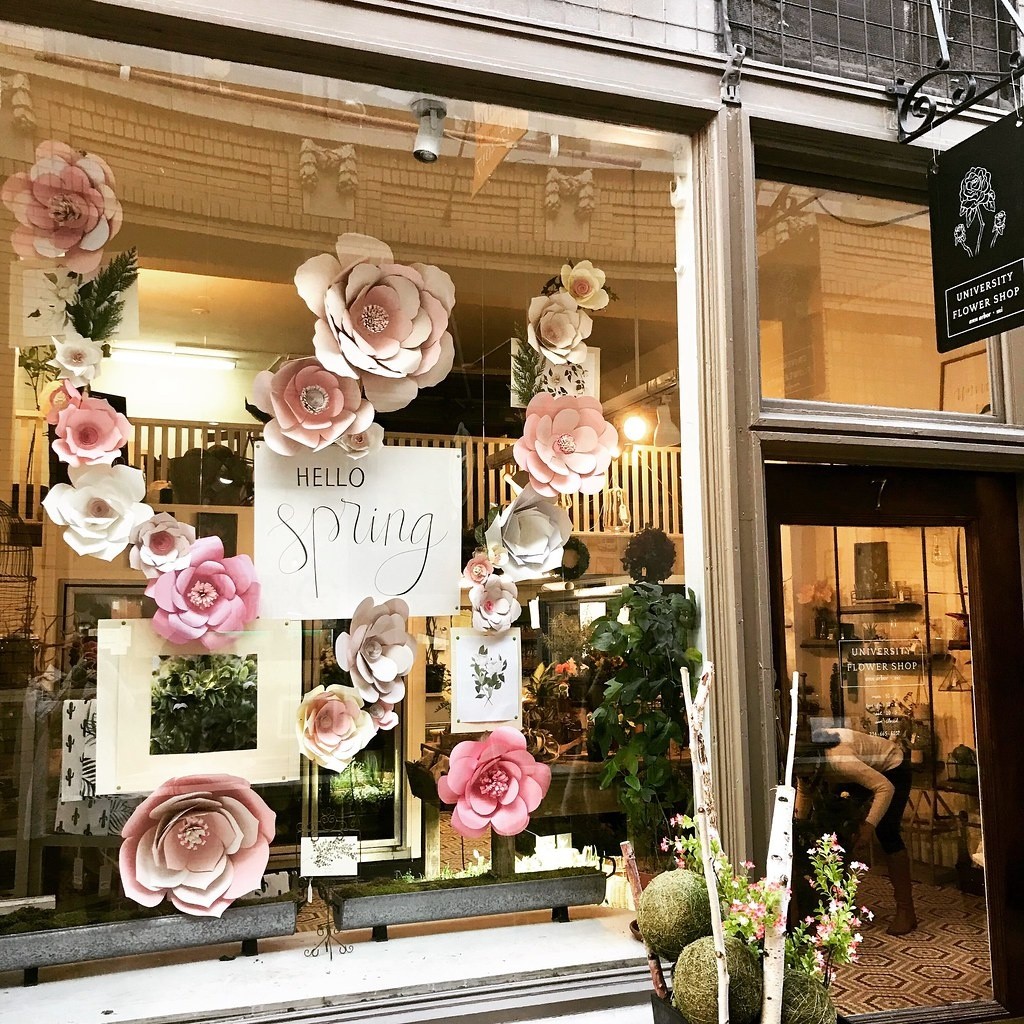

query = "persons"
[25,630,97,906]
[787,717,917,935]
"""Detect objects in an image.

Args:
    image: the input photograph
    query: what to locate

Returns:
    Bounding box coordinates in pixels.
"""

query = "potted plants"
[587,578,702,912]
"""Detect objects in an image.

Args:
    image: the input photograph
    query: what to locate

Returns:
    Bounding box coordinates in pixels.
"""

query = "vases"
[815,609,828,640]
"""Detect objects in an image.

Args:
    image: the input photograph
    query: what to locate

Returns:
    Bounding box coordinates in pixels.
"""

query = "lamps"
[411,98,449,162]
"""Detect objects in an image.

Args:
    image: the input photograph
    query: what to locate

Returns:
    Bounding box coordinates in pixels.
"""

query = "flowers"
[796,579,832,607]
[661,810,874,986]
[2,138,622,923]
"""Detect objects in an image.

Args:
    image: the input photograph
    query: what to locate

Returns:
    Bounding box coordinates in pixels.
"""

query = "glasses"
[792,763,802,778]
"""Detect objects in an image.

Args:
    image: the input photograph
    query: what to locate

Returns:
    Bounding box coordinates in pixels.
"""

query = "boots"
[885,849,917,934]
